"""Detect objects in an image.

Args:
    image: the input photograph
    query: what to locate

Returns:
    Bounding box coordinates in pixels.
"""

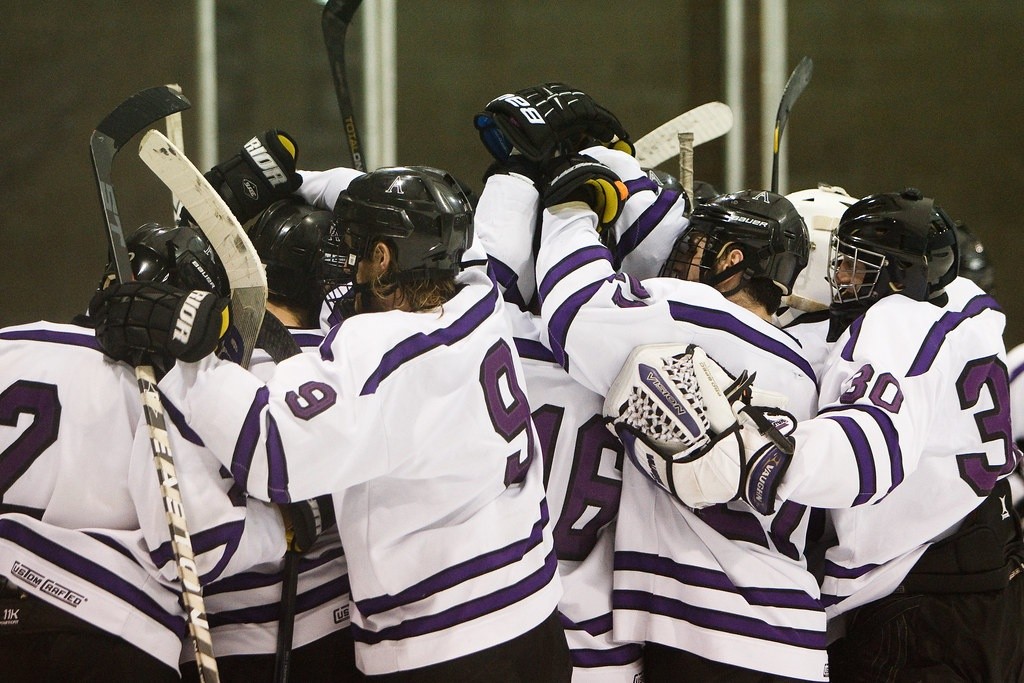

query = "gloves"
[280,492,335,556]
[179,129,305,226]
[89,281,231,363]
[474,82,636,226]
[601,341,797,517]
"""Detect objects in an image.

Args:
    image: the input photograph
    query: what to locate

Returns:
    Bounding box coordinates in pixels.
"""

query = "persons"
[602,189,1024,683]
[0,127,575,683]
[472,79,860,683]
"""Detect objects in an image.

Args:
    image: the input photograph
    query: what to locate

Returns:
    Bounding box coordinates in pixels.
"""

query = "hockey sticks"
[274,550,301,683]
[770,56,816,195]
[91,85,223,683]
[320,0,367,171]
[674,131,697,215]
[632,99,735,171]
[136,125,270,369]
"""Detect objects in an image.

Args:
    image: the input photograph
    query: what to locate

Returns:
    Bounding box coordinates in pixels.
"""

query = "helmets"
[780,186,860,306]
[949,220,995,303]
[245,195,334,307]
[656,191,810,298]
[827,188,959,319]
[319,165,474,290]
[117,223,230,299]
[679,180,720,218]
